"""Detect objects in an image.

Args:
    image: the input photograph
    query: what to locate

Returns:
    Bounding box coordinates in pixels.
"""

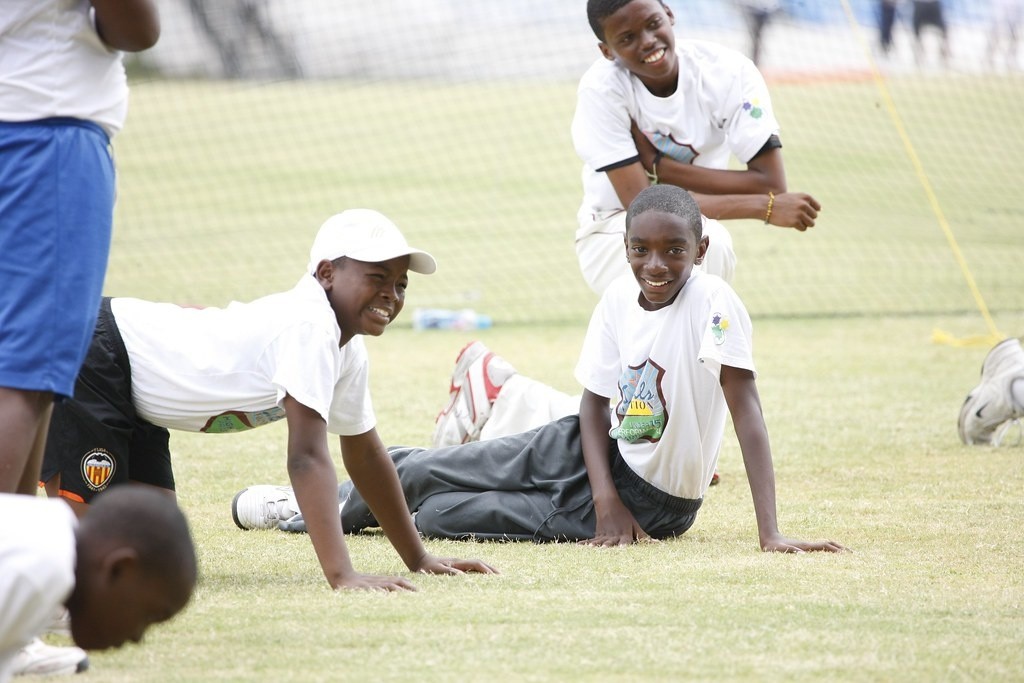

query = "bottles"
[411,307,493,332]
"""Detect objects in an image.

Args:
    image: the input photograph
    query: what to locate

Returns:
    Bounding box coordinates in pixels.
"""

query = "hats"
[309,210,438,275]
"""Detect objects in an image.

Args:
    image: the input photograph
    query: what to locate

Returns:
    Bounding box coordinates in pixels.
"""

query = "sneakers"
[432,340,519,450]
[232,480,354,534]
[958,337,1024,446]
[12,636,90,676]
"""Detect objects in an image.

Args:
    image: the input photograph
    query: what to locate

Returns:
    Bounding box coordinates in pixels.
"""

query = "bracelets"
[765,192,774,224]
[644,153,664,186]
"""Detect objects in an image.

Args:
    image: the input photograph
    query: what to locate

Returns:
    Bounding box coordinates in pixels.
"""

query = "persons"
[879,0,950,71]
[956,337,1024,446]
[0,0,822,683]
